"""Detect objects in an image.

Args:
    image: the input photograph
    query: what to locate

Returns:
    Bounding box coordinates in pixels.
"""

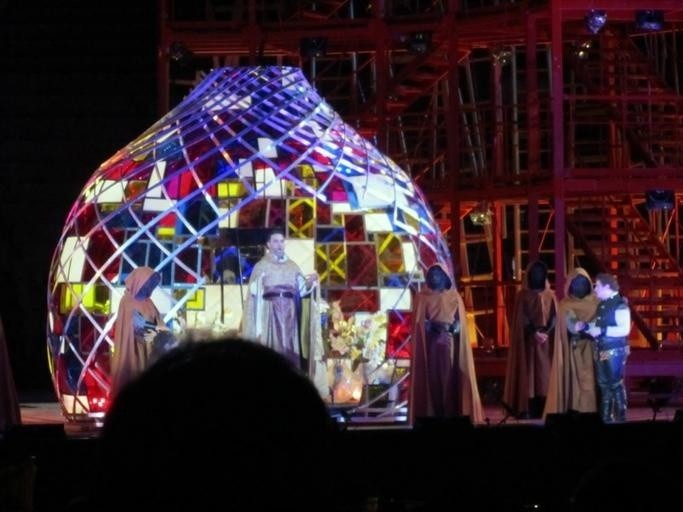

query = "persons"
[540,267,630,422]
[406,262,487,428]
[574,272,633,423]
[88,340,356,510]
[108,265,171,401]
[244,230,331,400]
[502,258,558,421]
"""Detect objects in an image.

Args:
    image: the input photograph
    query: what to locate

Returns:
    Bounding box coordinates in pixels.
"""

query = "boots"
[601,400,626,422]
